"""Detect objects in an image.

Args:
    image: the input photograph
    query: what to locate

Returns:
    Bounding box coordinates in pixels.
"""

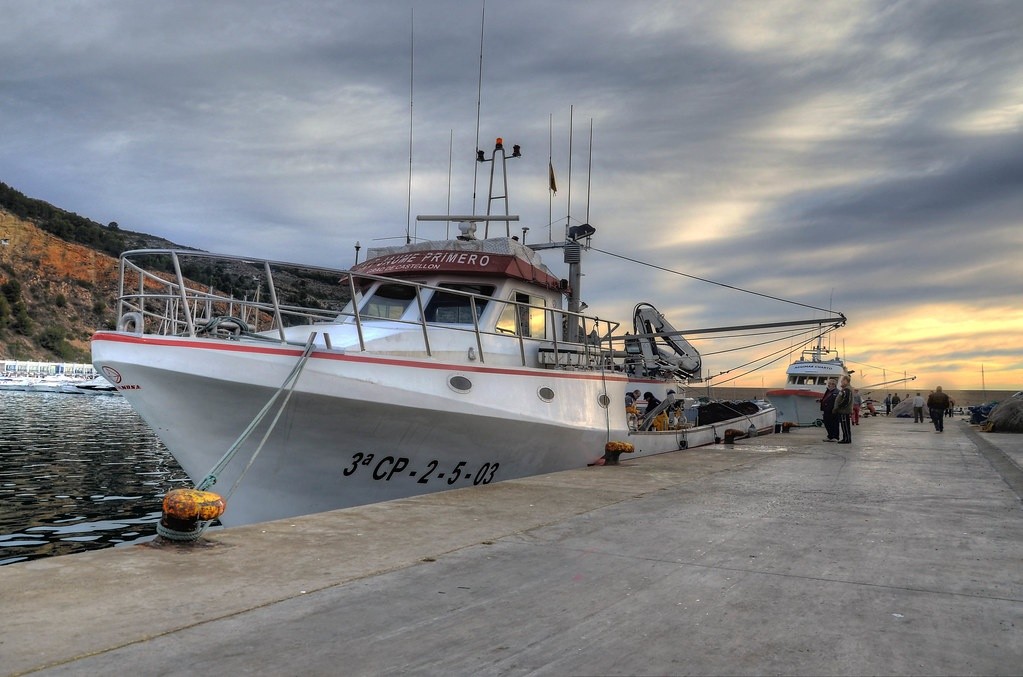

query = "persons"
[643,392,663,430]
[927,386,950,433]
[913,393,925,423]
[833,376,853,443]
[816,379,840,442]
[627,390,641,430]
[886,393,909,415]
[851,389,862,425]
[867,396,875,416]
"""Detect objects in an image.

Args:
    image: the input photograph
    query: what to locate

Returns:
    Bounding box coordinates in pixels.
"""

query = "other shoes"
[852,421,855,425]
[823,437,839,442]
[856,424,859,425]
[936,427,943,432]
[839,439,851,444]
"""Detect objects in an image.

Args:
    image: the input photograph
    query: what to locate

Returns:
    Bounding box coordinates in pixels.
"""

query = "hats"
[643,392,652,400]
